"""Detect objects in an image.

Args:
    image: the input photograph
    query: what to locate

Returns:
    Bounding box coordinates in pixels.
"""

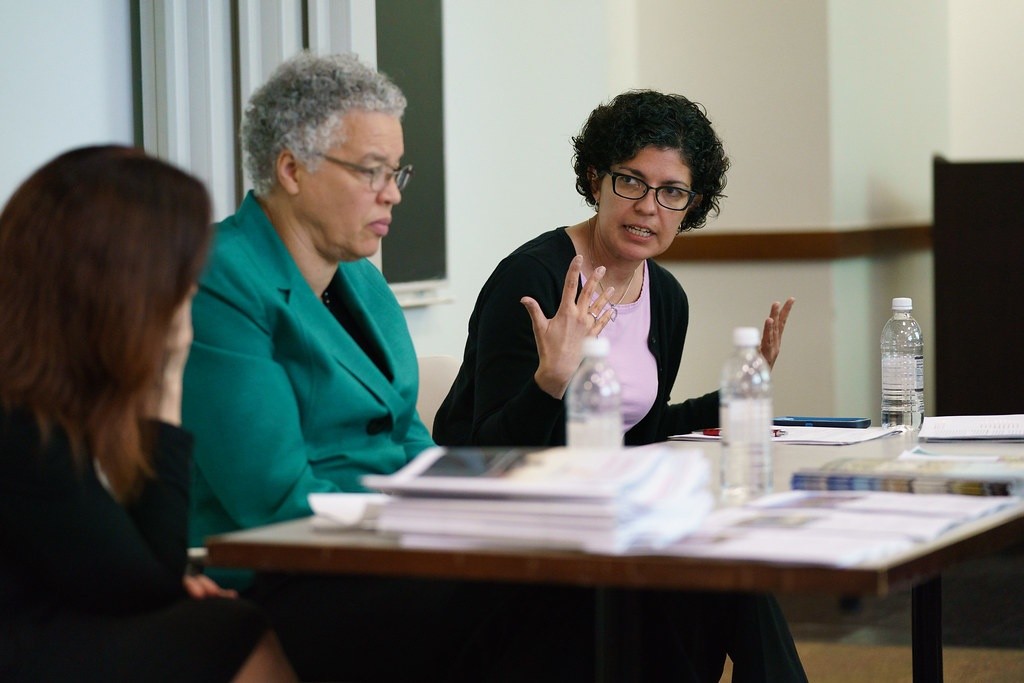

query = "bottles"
[719,326,774,496]
[880,298,925,436]
[565,337,622,480]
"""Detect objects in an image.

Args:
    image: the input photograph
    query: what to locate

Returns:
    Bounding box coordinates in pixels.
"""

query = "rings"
[588,312,597,320]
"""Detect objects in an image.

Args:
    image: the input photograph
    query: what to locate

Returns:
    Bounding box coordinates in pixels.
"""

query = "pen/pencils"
[691,427,786,438]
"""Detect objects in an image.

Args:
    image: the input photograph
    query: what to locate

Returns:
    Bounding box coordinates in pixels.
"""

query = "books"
[920,413,1024,443]
[361,446,714,556]
[793,458,1024,497]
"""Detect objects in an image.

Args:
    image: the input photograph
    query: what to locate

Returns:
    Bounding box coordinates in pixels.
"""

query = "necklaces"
[323,285,331,307]
[588,225,636,322]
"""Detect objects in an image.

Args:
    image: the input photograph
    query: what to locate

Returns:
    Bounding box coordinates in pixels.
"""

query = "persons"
[0,142,299,682]
[182,54,698,682]
[431,89,806,683]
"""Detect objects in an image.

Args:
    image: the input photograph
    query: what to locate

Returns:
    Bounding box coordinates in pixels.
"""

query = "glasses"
[317,151,414,193]
[605,167,700,211]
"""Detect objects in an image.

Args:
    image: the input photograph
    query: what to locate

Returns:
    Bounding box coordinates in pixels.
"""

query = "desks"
[206,414,1024,682]
